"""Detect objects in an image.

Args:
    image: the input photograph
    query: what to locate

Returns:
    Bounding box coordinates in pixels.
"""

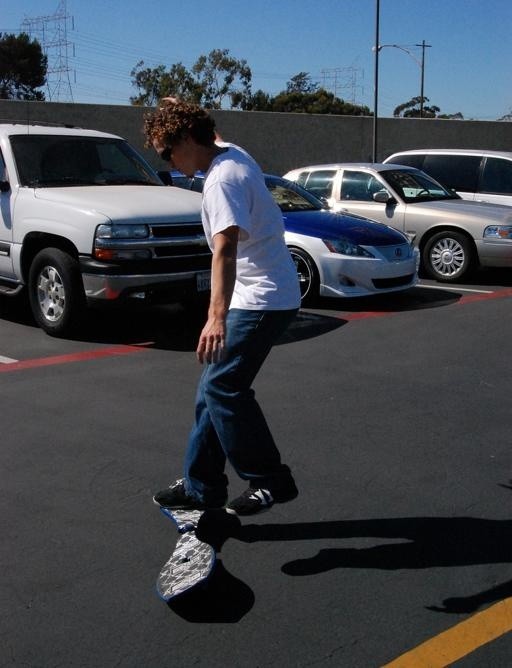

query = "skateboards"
[156,478,217,605]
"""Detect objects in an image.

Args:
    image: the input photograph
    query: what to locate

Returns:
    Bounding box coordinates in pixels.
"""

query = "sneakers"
[225,471,300,516]
[152,473,229,509]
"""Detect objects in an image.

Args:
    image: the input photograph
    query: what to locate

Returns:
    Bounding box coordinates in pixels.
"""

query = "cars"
[167,168,418,309]
[271,162,508,284]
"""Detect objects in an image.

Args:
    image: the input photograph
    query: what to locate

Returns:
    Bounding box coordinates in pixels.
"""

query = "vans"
[366,147,509,206]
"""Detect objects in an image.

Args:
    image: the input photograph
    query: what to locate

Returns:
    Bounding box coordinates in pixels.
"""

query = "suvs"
[1,126,212,336]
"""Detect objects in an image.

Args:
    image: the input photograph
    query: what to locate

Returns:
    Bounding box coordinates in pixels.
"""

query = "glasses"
[160,142,175,162]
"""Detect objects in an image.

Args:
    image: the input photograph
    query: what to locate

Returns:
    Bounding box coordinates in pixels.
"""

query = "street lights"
[370,35,432,120]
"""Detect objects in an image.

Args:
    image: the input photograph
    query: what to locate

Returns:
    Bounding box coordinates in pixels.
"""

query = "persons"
[144,98,304,518]
